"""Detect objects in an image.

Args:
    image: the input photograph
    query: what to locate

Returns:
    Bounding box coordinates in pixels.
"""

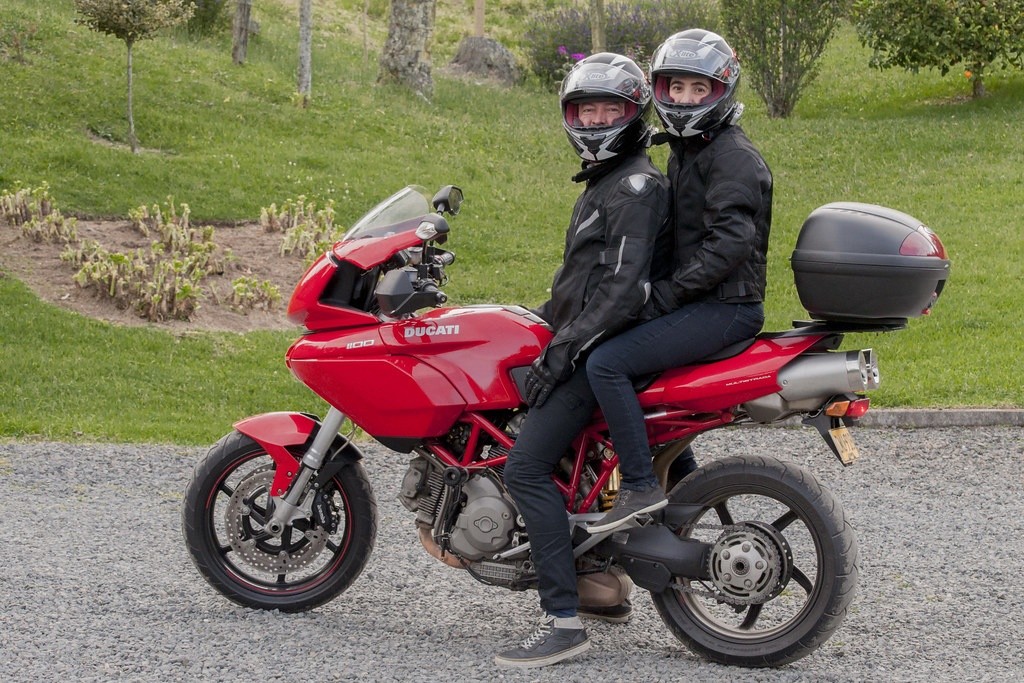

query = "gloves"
[523,343,569,410]
[633,297,663,324]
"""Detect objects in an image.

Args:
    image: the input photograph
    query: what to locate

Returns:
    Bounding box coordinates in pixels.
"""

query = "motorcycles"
[179,182,952,669]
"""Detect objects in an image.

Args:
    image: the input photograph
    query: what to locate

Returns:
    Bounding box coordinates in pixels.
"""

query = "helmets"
[558,52,650,160]
[649,27,741,140]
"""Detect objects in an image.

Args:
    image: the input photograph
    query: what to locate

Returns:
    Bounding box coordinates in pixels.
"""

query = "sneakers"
[494,611,590,667]
[587,485,669,533]
[576,600,635,622]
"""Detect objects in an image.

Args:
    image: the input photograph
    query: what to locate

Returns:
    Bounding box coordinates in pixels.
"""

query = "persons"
[583,28,774,535]
[493,51,675,671]
